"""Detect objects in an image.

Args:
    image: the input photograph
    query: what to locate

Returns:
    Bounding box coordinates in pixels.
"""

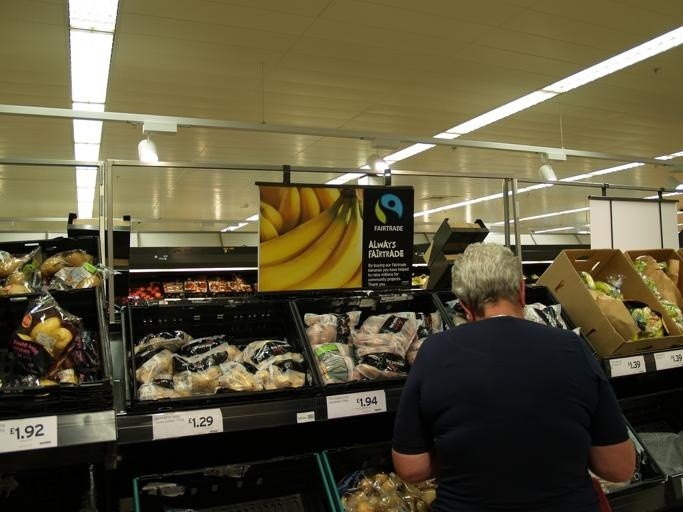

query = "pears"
[345,474,436,512]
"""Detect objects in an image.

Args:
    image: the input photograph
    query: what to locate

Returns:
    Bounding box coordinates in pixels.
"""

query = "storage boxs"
[0,233,683,512]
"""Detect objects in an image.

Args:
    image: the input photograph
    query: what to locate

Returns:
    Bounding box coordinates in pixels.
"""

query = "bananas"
[260,188,364,291]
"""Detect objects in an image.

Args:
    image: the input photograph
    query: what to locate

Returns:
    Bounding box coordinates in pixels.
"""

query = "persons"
[388,239,639,511]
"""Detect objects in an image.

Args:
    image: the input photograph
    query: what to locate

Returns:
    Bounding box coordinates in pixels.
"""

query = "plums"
[131,287,162,300]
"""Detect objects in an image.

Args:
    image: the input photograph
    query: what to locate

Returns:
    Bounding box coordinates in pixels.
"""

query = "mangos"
[18,316,73,355]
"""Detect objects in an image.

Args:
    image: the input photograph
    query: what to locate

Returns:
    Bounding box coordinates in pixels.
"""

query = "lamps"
[138,122,179,162]
[539,155,567,185]
[667,165,683,191]
[368,139,401,177]
